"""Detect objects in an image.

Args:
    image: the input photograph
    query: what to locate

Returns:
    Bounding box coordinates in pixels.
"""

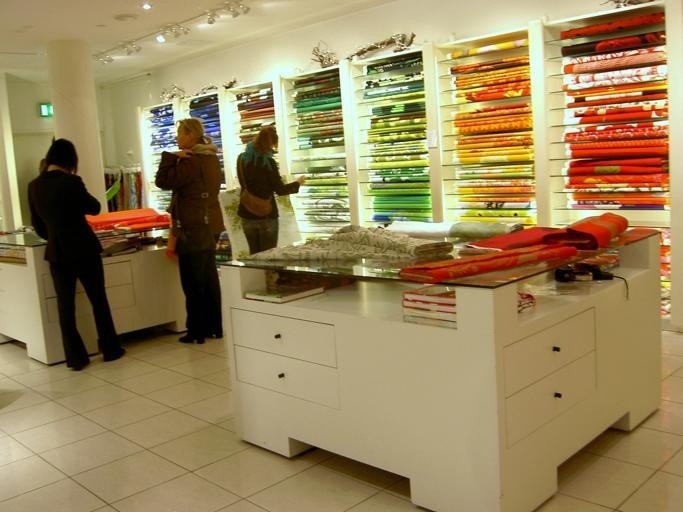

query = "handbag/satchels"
[175,223,216,253]
[239,189,272,217]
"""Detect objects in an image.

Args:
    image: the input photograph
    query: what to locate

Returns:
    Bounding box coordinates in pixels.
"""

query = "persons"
[238,126,307,254]
[154,117,224,344]
[26,138,127,371]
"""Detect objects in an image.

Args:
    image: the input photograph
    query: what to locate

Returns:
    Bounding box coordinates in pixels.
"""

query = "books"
[240,287,326,305]
[400,283,461,331]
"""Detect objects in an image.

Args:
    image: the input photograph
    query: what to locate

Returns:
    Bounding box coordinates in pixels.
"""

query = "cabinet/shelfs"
[2,227,186,366]
[212,226,662,512]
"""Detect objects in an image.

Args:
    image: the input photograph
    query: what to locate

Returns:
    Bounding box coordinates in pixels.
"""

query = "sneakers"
[103,349,124,361]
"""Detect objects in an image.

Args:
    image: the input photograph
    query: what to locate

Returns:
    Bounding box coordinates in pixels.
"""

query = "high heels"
[179,333,204,343]
[204,332,221,338]
